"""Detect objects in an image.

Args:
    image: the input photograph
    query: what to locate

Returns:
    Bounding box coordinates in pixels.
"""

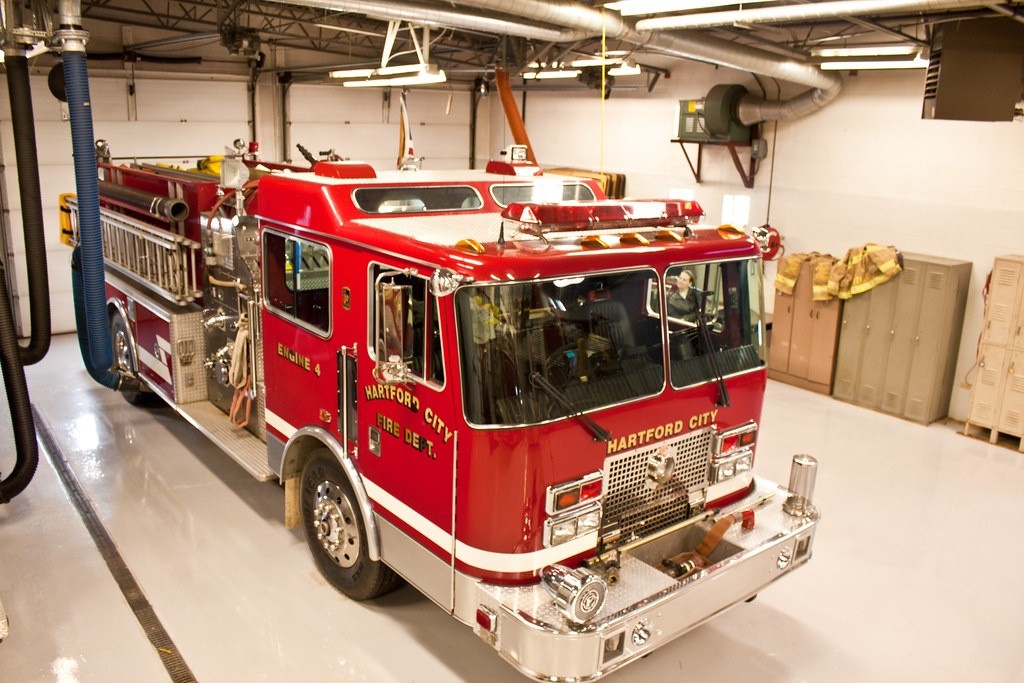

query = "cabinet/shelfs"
[965,254,1024,452]
[834,247,973,427]
[767,253,846,395]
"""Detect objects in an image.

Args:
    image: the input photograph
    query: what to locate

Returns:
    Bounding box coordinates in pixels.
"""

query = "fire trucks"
[56,134,821,683]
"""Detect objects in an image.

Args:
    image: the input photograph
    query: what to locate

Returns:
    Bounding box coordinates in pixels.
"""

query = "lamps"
[329,21,448,88]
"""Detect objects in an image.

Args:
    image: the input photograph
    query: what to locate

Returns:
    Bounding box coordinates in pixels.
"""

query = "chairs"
[581,300,636,349]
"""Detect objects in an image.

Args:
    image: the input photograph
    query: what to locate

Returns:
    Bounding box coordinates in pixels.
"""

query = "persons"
[667,270,700,321]
